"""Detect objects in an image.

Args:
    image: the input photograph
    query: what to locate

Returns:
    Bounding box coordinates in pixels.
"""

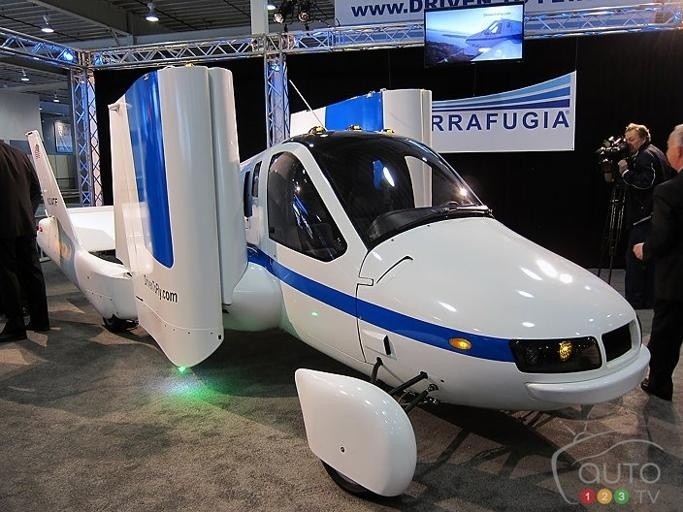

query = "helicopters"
[465,12,523,48]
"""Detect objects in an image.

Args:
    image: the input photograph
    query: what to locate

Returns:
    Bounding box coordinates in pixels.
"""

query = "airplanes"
[26,64,650,500]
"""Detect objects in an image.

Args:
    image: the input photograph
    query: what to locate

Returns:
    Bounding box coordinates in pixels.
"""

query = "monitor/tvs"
[424,1,524,69]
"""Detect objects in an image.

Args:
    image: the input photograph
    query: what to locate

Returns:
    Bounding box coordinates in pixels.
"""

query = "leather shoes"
[0,318,49,343]
[640,378,673,401]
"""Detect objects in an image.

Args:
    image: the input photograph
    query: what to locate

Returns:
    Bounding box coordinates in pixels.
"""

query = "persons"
[0,139,50,343]
[618,123,683,401]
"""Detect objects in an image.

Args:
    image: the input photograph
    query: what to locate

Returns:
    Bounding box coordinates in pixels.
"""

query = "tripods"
[596,172,633,285]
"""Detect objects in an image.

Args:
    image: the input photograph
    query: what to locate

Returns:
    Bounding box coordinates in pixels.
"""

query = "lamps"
[145,2,159,22]
[39,15,53,34]
[273,0,315,37]
